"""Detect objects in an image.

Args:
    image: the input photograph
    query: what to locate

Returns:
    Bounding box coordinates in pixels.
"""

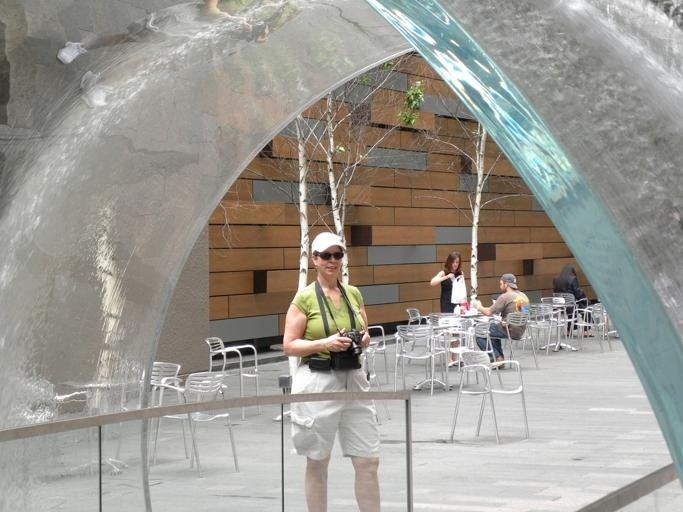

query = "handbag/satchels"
[308,356,332,372]
[450,274,468,304]
[329,340,362,370]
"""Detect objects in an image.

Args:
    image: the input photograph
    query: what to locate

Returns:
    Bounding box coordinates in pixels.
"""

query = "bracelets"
[476,305,481,312]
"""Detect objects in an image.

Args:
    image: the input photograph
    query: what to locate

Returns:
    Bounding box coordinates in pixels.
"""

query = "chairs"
[205,335,261,417]
[367,291,621,443]
[148,361,239,472]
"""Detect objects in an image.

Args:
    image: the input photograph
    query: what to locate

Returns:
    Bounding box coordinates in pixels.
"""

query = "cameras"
[341,328,365,357]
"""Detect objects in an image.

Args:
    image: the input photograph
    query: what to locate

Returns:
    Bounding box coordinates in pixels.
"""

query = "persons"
[430,252,469,369]
[553,264,594,337]
[471,272,531,369]
[283,232,380,512]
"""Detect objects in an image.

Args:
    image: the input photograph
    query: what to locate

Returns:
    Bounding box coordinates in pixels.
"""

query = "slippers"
[566,334,570,336]
[583,333,595,337]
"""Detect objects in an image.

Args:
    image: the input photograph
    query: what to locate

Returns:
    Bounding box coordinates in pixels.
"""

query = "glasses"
[313,250,344,260]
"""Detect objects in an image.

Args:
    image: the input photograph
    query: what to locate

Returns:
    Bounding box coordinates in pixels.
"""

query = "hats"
[498,272,519,290]
[311,231,345,254]
